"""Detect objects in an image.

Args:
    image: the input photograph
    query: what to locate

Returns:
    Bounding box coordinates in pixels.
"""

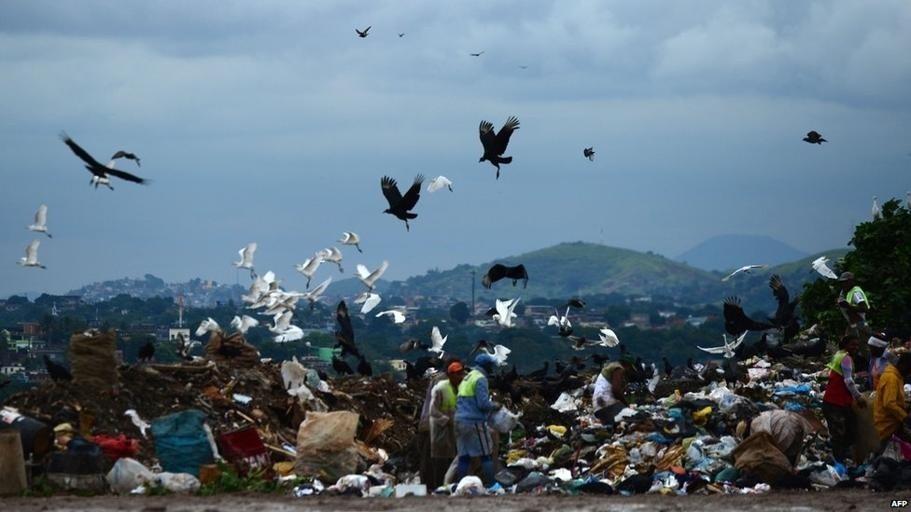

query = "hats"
[618,352,634,365]
[473,353,498,367]
[446,362,468,375]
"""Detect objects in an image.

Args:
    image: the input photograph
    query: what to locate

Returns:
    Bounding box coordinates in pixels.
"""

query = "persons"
[868,331,890,391]
[736,409,816,467]
[429,362,465,493]
[46,423,102,474]
[418,366,448,432]
[823,335,868,487]
[830,272,871,337]
[592,353,639,428]
[896,340,911,357]
[453,353,504,488]
[873,353,911,448]
[722,358,746,389]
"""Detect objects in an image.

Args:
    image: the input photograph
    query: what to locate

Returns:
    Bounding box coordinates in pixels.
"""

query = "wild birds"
[60,133,148,192]
[170,226,874,380]
[380,172,426,232]
[135,333,155,364]
[355,25,372,37]
[802,129,827,145]
[469,50,486,57]
[870,195,880,222]
[616,463,659,495]
[39,352,74,386]
[13,203,54,272]
[426,176,454,193]
[478,115,521,181]
[583,146,596,163]
